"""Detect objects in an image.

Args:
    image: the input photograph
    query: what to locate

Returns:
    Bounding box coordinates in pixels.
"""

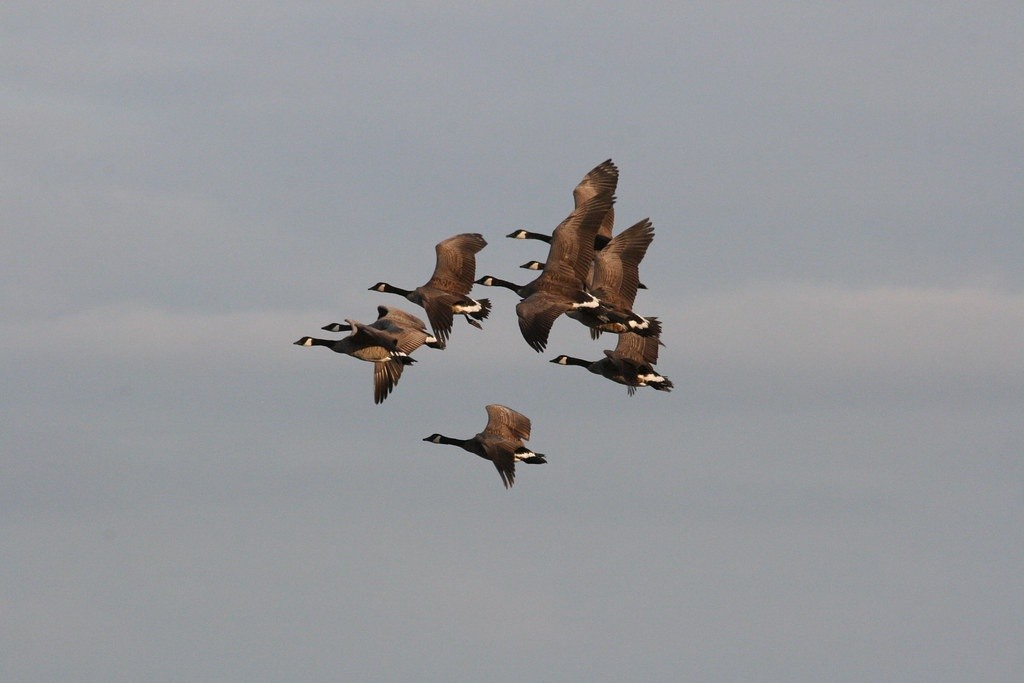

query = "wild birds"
[422,404,547,490]
[472,188,615,353]
[368,233,493,349]
[321,305,445,355]
[549,317,674,397]
[293,319,419,405]
[505,159,663,340]
